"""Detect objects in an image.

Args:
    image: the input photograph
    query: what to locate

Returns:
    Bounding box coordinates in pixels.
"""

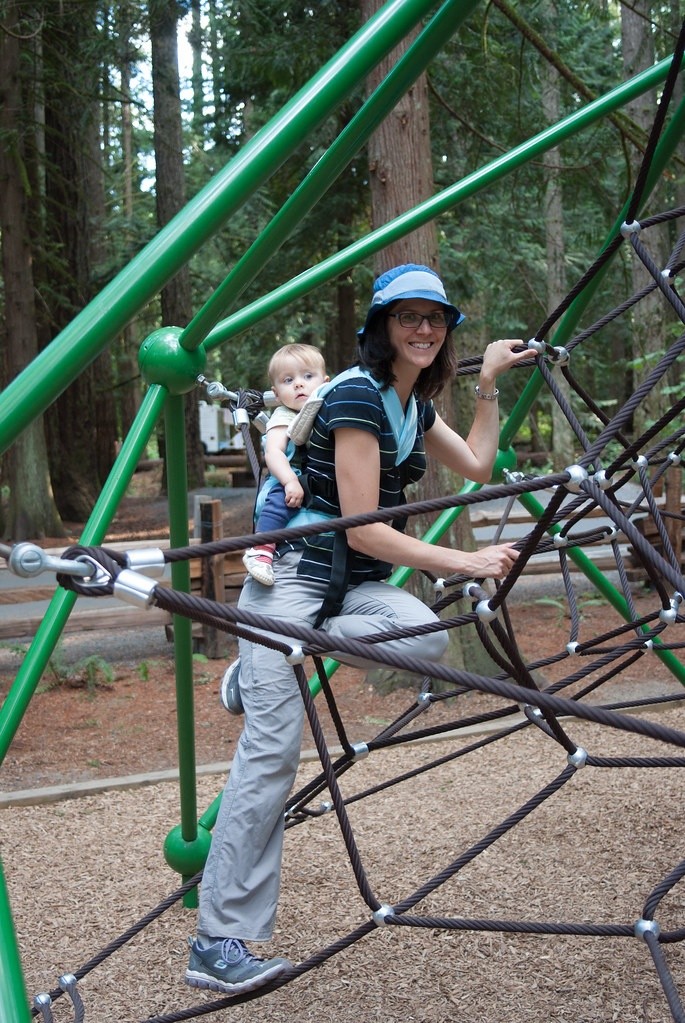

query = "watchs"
[475,386,499,400]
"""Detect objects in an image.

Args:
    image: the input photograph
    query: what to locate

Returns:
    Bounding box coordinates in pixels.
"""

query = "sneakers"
[184,936,291,995]
[220,656,244,715]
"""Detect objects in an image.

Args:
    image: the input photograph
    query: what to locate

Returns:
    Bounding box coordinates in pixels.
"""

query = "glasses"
[387,313,453,328]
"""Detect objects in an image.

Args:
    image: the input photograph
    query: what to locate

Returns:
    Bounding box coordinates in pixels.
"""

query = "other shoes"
[242,547,275,586]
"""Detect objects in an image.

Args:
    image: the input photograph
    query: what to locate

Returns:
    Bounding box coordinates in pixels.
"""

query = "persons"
[182,264,538,994]
[242,344,330,586]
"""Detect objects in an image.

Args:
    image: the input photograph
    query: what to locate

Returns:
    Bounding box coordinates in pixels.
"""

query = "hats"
[356,264,466,340]
